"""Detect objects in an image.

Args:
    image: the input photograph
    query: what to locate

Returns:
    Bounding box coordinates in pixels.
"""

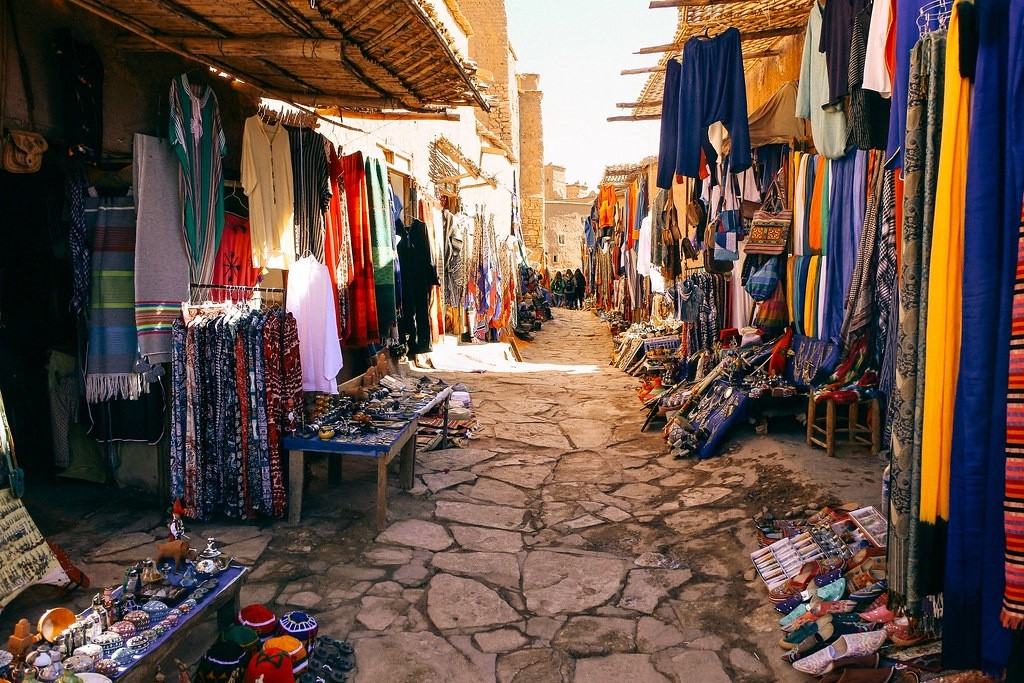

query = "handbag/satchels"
[661,154,793,333]
[1,127,49,175]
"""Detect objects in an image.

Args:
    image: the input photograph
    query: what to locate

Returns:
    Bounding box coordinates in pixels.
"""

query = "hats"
[200,605,318,683]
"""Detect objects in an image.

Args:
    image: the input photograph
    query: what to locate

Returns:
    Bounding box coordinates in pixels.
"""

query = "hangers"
[224,179,249,220]
[325,120,339,145]
[694,10,730,37]
[683,264,703,287]
[672,53,682,60]
[400,214,416,227]
[300,229,313,257]
[93,159,133,192]
[186,56,207,96]
[182,284,284,316]
[257,90,280,133]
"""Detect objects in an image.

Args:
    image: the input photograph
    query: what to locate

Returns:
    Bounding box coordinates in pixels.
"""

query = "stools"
[807,389,880,457]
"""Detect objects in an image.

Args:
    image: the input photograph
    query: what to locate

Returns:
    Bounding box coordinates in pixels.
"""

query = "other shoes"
[766,547,942,676]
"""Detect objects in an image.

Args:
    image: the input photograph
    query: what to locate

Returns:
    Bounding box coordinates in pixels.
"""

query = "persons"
[573,269,586,310]
[564,269,577,309]
[168,510,185,543]
[550,271,565,307]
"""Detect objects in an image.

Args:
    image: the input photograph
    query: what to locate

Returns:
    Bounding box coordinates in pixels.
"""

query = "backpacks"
[565,276,575,292]
[576,277,584,288]
[554,282,562,292]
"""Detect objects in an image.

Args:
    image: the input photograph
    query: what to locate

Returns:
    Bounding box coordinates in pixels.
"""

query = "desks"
[282,406,420,532]
[0,558,249,683]
[412,385,454,489]
